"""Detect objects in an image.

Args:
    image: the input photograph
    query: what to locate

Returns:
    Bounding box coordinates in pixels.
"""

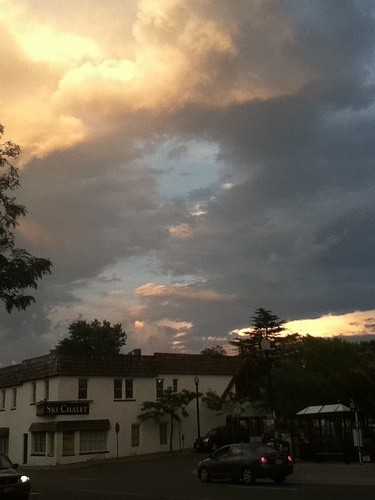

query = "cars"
[197,443,294,485]
[193,424,247,452]
[0,453,31,500]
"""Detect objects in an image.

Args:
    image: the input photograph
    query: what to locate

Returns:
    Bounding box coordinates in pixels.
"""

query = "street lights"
[194,375,200,438]
